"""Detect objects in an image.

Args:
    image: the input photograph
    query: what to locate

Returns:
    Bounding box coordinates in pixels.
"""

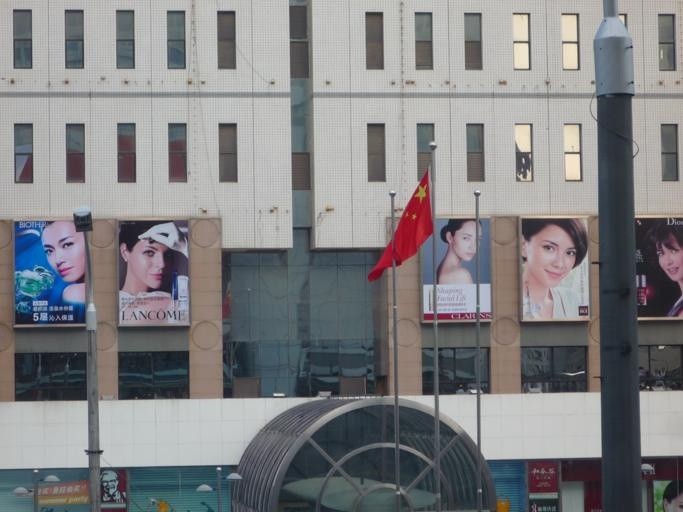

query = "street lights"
[73,210,101,511]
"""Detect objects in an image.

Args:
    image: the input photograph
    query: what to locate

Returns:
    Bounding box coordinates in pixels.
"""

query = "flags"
[366,162,433,284]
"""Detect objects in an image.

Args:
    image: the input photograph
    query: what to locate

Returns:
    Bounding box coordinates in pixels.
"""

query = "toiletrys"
[176,275,189,312]
[17,270,44,297]
[169,270,180,312]
[635,274,648,306]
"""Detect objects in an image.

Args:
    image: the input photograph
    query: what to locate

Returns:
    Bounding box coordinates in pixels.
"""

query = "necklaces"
[528,294,551,311]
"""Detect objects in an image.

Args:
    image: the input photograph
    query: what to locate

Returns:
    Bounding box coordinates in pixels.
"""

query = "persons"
[98,468,128,507]
[118,219,189,327]
[642,218,682,319]
[38,220,87,306]
[520,216,589,321]
[435,218,483,285]
[661,480,682,511]
